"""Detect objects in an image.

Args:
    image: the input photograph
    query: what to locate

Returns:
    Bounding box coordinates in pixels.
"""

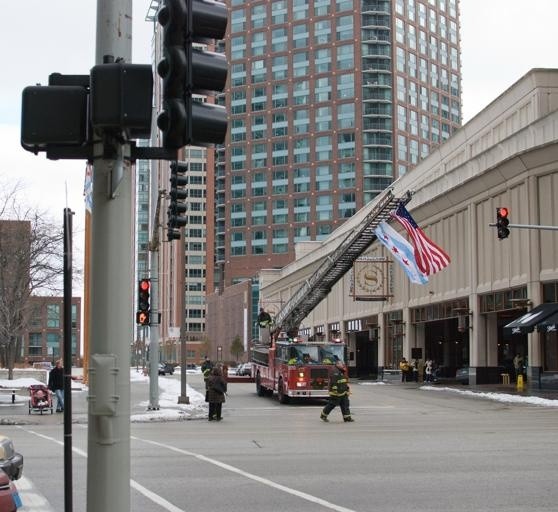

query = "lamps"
[391,319,405,340]
[453,308,473,332]
[316,330,339,342]
[367,323,380,342]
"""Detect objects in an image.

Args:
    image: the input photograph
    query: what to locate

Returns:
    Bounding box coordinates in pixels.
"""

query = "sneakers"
[344,419,354,422]
[320,413,329,422]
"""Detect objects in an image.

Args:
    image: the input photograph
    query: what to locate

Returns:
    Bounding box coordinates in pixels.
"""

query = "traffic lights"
[496,206,509,240]
[137,278,151,312]
[154,0,235,160]
[164,157,193,245]
[91,63,152,134]
[135,310,150,325]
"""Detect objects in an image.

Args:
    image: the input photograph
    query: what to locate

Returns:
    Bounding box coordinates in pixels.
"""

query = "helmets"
[335,360,347,371]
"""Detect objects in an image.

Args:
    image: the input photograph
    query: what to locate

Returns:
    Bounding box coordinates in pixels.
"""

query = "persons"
[398,357,438,383]
[49,359,68,412]
[320,361,354,423]
[199,355,228,421]
[512,353,528,375]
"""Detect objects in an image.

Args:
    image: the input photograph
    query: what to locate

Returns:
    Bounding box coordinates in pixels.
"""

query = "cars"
[227,359,253,377]
[159,361,174,376]
[0,434,26,484]
[0,467,23,512]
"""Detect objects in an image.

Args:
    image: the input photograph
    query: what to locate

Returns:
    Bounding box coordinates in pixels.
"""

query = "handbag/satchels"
[213,382,225,393]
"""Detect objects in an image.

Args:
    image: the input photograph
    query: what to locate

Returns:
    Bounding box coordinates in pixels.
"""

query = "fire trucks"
[251,186,415,405]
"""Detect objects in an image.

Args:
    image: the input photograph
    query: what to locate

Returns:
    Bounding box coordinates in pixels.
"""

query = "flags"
[371,224,430,285]
[390,202,451,273]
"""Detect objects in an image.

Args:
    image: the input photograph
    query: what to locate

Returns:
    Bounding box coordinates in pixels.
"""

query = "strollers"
[26,382,56,417]
